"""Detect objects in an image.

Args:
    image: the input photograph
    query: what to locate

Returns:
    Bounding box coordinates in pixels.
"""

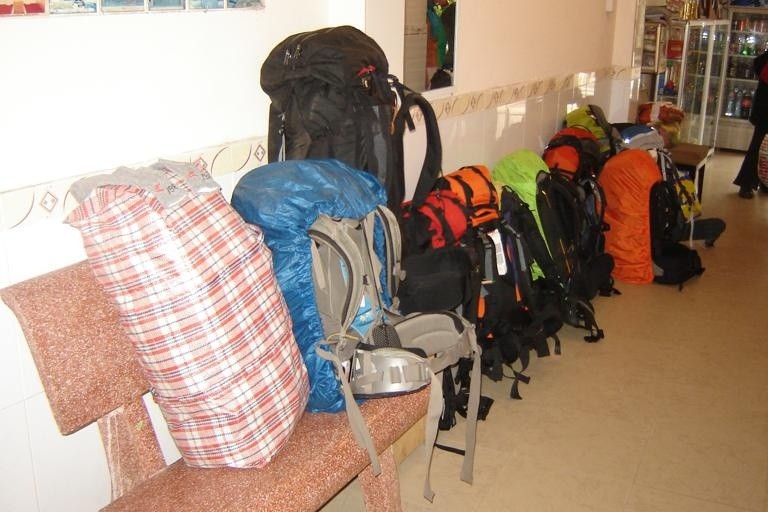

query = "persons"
[732,45,768,199]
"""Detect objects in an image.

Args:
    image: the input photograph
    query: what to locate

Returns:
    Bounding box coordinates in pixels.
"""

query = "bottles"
[725,17,768,119]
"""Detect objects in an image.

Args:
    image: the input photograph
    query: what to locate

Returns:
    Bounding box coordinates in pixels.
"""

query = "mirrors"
[403,0,457,96]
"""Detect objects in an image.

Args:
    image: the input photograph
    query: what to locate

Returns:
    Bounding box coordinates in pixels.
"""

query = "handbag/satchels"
[64,159,313,469]
[757,134,768,188]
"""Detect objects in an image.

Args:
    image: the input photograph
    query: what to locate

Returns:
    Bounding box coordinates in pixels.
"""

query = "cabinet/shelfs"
[639,4,768,151]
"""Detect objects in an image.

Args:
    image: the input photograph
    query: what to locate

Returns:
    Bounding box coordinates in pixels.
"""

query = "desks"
[666,143,714,205]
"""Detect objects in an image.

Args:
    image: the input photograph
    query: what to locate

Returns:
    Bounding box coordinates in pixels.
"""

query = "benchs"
[0,260,429,511]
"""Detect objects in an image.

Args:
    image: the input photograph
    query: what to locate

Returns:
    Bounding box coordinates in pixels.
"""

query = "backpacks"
[260,25,444,256]
[229,158,477,414]
[400,99,701,400]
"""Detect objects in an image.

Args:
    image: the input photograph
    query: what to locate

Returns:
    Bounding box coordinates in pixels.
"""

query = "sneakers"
[737,186,754,198]
